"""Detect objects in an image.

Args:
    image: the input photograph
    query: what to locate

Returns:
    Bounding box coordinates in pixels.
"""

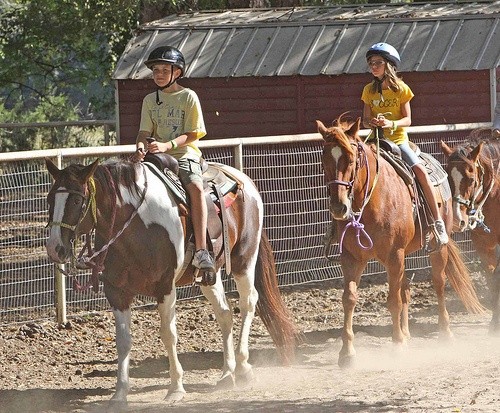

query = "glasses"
[368,60,385,67]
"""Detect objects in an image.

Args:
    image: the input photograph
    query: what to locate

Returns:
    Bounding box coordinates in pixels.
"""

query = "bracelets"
[389,122,397,131]
[171,139,177,150]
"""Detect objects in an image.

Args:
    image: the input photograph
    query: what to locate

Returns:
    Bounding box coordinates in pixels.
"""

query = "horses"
[43,154,300,410]
[439,127,500,331]
[315,110,490,366]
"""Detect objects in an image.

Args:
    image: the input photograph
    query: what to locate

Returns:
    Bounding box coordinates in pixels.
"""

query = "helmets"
[366,43,400,70]
[144,46,185,79]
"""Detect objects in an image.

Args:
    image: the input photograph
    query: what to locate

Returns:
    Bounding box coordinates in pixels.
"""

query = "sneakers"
[435,221,448,245]
[191,251,214,270]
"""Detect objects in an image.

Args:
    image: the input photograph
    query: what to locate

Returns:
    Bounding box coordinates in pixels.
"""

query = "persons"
[75,46,215,272]
[324,42,450,246]
